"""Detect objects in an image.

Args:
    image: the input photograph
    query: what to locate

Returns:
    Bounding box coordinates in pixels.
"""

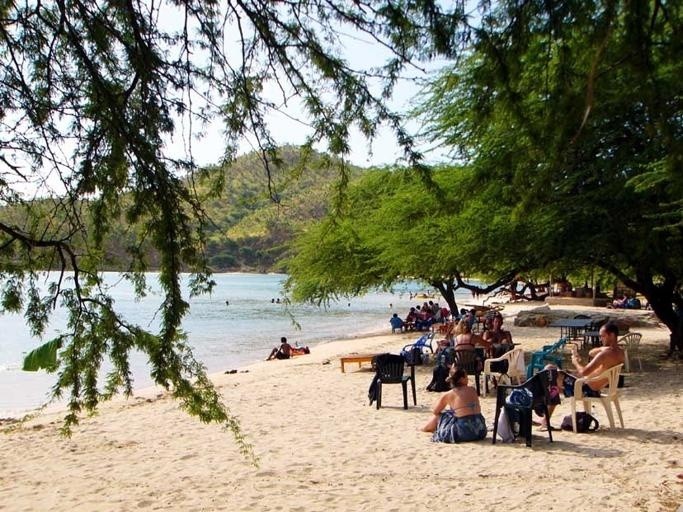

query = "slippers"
[536,424,554,431]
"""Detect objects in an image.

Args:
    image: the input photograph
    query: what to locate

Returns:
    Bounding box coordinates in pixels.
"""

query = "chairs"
[370,307,641,448]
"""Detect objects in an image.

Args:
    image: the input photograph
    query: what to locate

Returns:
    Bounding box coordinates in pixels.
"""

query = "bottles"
[590,405,600,430]
[424,350,430,368]
[431,326,435,337]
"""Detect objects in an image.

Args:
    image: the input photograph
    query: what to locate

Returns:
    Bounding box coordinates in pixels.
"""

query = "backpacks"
[426,364,451,392]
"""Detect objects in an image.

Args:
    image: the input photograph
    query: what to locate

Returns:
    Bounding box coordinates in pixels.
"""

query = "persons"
[291,348,309,355]
[265,337,294,360]
[390,301,625,444]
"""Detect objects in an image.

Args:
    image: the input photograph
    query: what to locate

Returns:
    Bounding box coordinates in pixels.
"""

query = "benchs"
[340,353,381,372]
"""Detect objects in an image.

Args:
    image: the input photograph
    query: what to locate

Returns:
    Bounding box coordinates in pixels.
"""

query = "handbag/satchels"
[409,344,422,365]
[561,411,599,433]
